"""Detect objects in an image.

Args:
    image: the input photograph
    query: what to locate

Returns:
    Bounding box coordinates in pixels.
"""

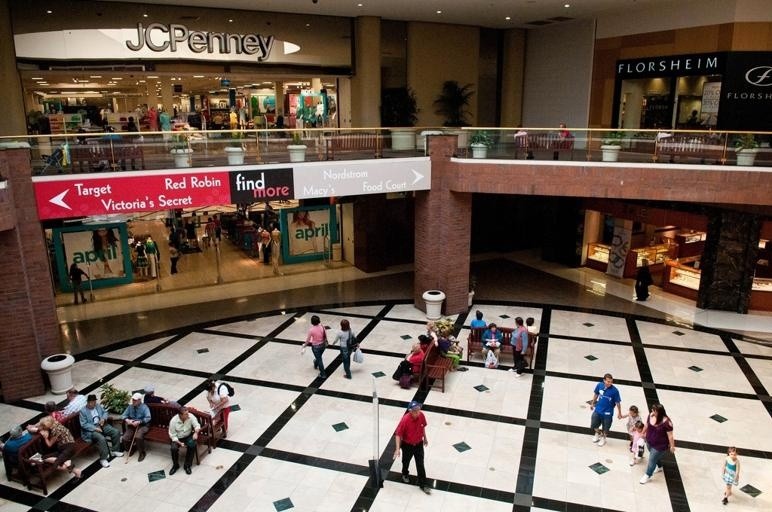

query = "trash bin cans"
[332,243,342,261]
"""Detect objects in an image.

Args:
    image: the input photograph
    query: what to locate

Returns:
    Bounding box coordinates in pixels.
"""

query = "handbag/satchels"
[353,348,363,363]
[347,337,358,350]
[399,374,411,389]
[516,336,523,351]
[485,350,498,369]
[323,331,329,347]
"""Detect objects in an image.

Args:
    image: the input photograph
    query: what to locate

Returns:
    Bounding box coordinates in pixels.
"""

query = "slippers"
[73,473,84,485]
[68,464,76,474]
[457,367,469,372]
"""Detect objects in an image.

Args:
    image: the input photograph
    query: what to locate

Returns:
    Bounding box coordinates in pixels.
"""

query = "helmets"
[408,400,422,411]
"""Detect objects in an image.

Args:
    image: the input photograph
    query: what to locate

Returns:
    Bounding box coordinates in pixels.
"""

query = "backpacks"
[217,382,234,397]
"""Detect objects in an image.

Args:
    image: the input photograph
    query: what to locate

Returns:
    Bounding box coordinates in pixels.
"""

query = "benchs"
[652,134,729,165]
[3,402,227,496]
[409,327,535,393]
[515,133,575,160]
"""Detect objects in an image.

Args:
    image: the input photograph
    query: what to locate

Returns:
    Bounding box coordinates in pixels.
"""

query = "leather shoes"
[138,451,146,461]
[184,463,192,474]
[129,446,136,457]
[169,463,180,475]
[420,485,431,493]
[402,472,410,482]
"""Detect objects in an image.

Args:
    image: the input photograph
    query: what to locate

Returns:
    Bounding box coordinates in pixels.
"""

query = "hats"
[144,384,155,395]
[131,392,142,401]
[86,394,98,402]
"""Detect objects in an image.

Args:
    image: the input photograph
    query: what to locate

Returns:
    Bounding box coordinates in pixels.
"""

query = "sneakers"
[640,473,653,484]
[100,458,110,468]
[722,497,728,505]
[509,368,525,378]
[318,372,326,378]
[654,465,663,473]
[630,458,642,465]
[313,359,318,369]
[724,491,733,496]
[344,374,352,379]
[111,449,124,457]
[592,431,607,447]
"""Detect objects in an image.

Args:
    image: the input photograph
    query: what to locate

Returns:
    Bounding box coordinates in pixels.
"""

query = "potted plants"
[286,132,308,163]
[432,79,477,147]
[600,133,623,162]
[167,132,193,168]
[224,132,247,164]
[470,130,493,159]
[379,84,422,151]
[735,133,760,166]
